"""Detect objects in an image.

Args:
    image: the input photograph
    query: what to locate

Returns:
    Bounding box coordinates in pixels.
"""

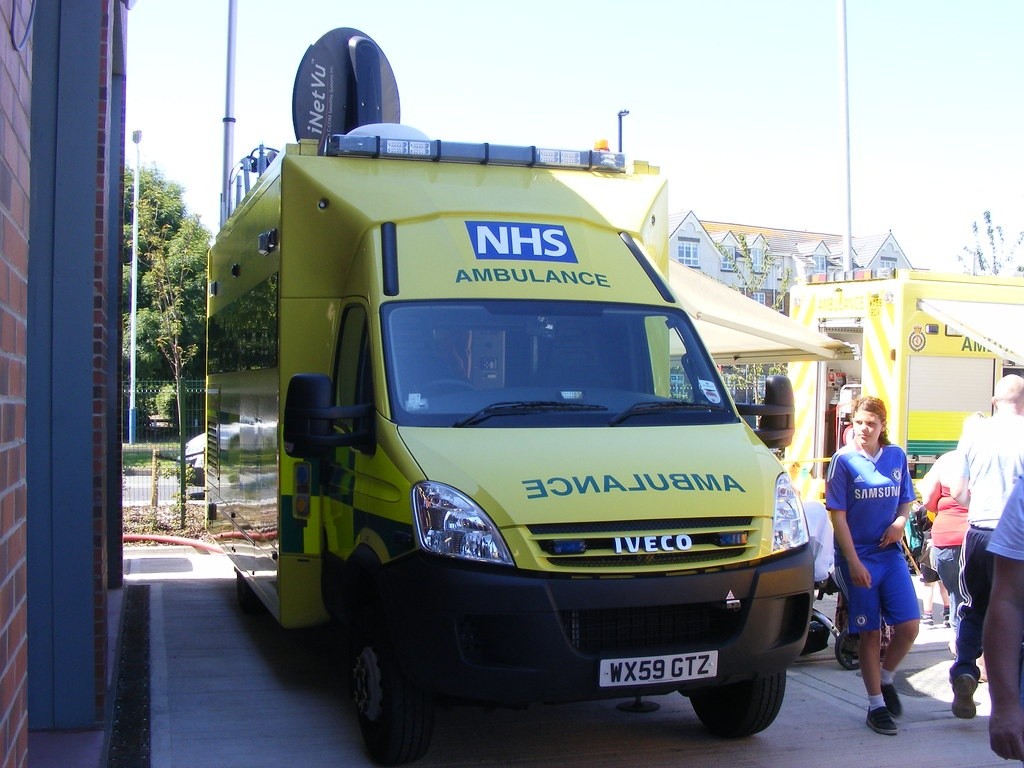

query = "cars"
[177,435,208,489]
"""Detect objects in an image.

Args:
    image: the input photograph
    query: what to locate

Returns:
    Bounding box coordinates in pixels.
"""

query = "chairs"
[404,350,473,399]
[547,338,614,387]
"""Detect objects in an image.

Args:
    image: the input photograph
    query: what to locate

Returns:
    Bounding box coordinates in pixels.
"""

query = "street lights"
[129,130,143,444]
[617,109,630,152]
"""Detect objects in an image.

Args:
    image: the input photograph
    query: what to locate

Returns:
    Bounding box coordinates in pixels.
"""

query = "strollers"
[801,501,862,670]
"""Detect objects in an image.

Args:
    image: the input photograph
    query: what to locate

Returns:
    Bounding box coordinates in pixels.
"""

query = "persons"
[921,413,985,660]
[983,470,1024,768]
[950,374,1024,719]
[825,396,921,735]
[913,500,951,630]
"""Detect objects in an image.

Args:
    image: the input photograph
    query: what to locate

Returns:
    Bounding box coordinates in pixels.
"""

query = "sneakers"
[943,610,950,625]
[952,672,976,720]
[882,683,903,718]
[865,708,898,734]
[923,611,933,625]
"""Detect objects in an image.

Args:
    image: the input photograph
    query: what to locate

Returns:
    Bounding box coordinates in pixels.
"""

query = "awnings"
[668,255,854,365]
[917,300,1024,367]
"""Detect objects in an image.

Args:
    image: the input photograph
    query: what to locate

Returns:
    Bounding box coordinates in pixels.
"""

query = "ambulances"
[783,267,1024,524]
[203,133,817,768]
